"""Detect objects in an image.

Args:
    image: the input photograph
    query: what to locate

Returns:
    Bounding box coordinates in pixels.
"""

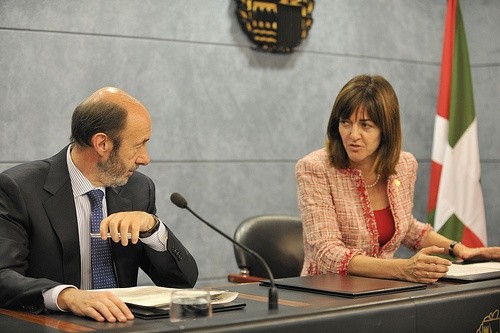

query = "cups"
[169,288,213,322]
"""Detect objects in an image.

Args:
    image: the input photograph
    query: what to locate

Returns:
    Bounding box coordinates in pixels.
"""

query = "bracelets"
[449,241,457,257]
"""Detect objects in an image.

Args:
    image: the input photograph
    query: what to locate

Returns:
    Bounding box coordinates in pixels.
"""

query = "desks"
[0,260,500,333]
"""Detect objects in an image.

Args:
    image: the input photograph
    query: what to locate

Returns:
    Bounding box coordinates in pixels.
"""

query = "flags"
[426,0,487,261]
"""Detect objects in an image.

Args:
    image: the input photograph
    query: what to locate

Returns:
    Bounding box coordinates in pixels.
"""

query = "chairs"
[228,214,305,283]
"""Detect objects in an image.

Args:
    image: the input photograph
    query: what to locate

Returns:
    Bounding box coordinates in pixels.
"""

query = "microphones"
[170,192,279,309]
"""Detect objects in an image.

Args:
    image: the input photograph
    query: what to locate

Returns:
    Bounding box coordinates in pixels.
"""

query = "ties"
[88,189,118,289]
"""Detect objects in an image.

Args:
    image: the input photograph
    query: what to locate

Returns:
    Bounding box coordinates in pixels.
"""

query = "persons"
[296,75,500,283]
[0,86,199,322]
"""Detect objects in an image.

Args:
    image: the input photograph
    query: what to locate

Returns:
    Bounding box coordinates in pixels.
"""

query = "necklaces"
[365,174,380,188]
